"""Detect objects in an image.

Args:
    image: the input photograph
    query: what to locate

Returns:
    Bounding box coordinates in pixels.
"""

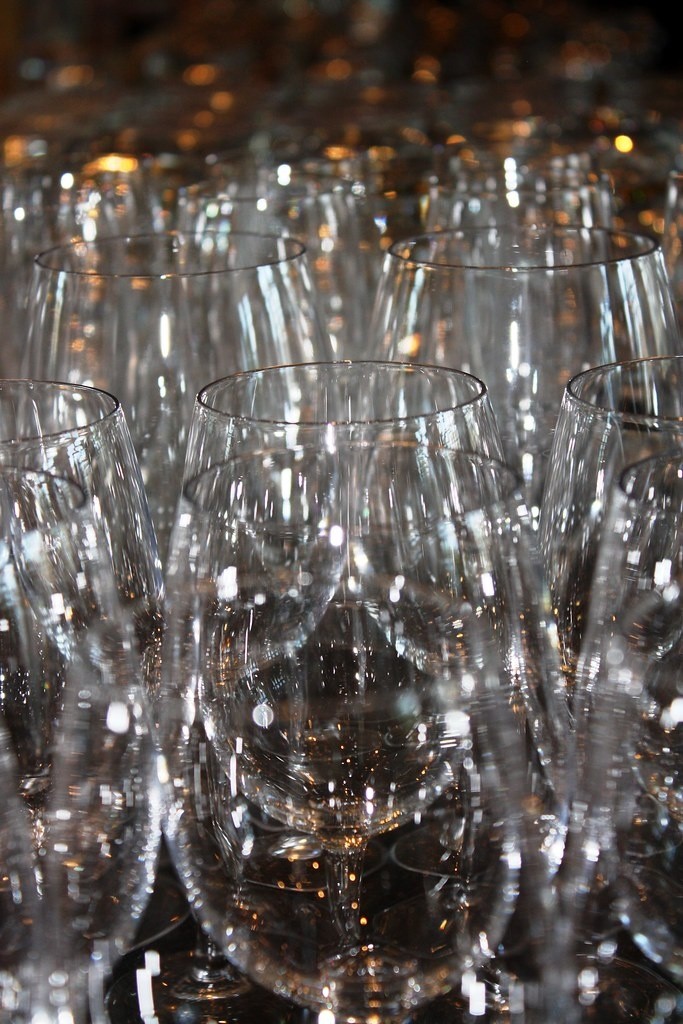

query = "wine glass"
[0,1,682,1024]
